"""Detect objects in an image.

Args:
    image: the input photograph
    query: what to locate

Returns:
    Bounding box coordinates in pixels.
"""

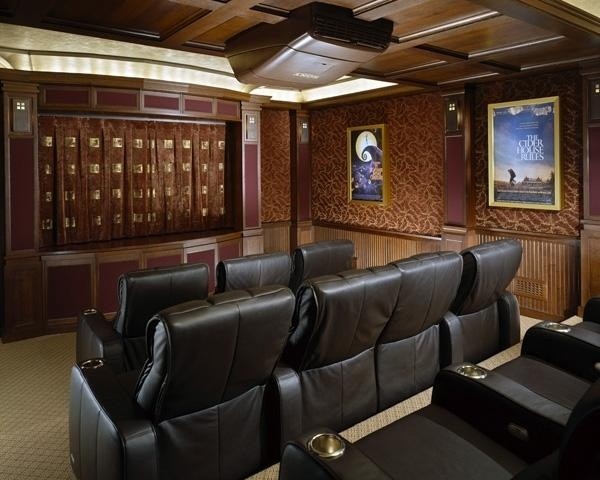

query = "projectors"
[224,1,394,91]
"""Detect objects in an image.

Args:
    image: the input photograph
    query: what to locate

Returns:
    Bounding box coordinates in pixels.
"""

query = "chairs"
[431,319,600,464]
[291,239,357,296]
[521,296,600,382]
[279,361,600,480]
[375,251,466,412]
[76,262,209,367]
[269,264,402,460]
[68,284,306,480]
[442,240,523,373]
[212,250,291,296]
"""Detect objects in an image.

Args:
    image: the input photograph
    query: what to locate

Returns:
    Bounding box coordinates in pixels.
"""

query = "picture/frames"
[346,124,389,207]
[487,95,561,211]
